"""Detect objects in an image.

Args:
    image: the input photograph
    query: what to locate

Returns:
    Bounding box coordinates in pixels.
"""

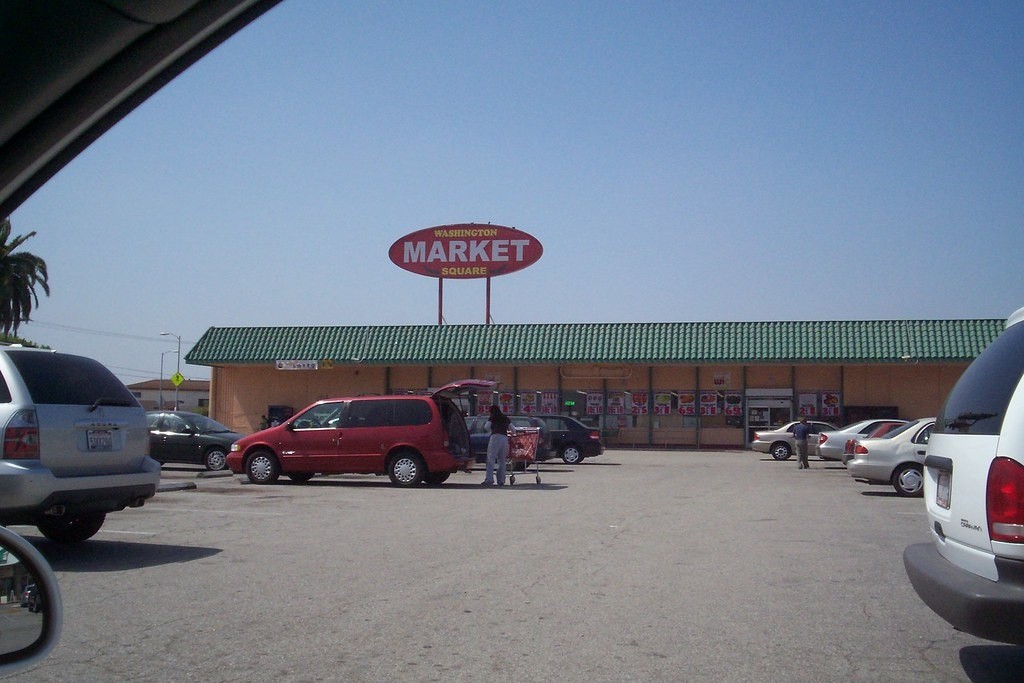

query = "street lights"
[159,349,179,409]
[158,331,182,411]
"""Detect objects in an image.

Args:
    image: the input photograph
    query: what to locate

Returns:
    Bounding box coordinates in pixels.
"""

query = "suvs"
[902,304,1024,645]
[0,339,163,541]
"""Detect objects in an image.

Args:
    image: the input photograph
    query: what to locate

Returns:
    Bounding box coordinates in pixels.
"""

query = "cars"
[846,415,939,497]
[751,419,839,461]
[141,410,247,472]
[21,583,41,607]
[814,418,911,466]
[463,413,606,465]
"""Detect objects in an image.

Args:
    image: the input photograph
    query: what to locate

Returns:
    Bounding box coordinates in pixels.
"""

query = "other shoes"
[481,481,493,486]
[498,481,504,486]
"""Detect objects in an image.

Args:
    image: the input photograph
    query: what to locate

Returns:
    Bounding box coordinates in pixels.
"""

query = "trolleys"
[501,426,541,485]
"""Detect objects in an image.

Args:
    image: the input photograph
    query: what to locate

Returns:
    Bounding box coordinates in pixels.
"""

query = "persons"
[481,406,515,486]
[793,417,810,469]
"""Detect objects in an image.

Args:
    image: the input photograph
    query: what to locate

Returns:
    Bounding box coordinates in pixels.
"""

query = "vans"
[226,379,501,487]
